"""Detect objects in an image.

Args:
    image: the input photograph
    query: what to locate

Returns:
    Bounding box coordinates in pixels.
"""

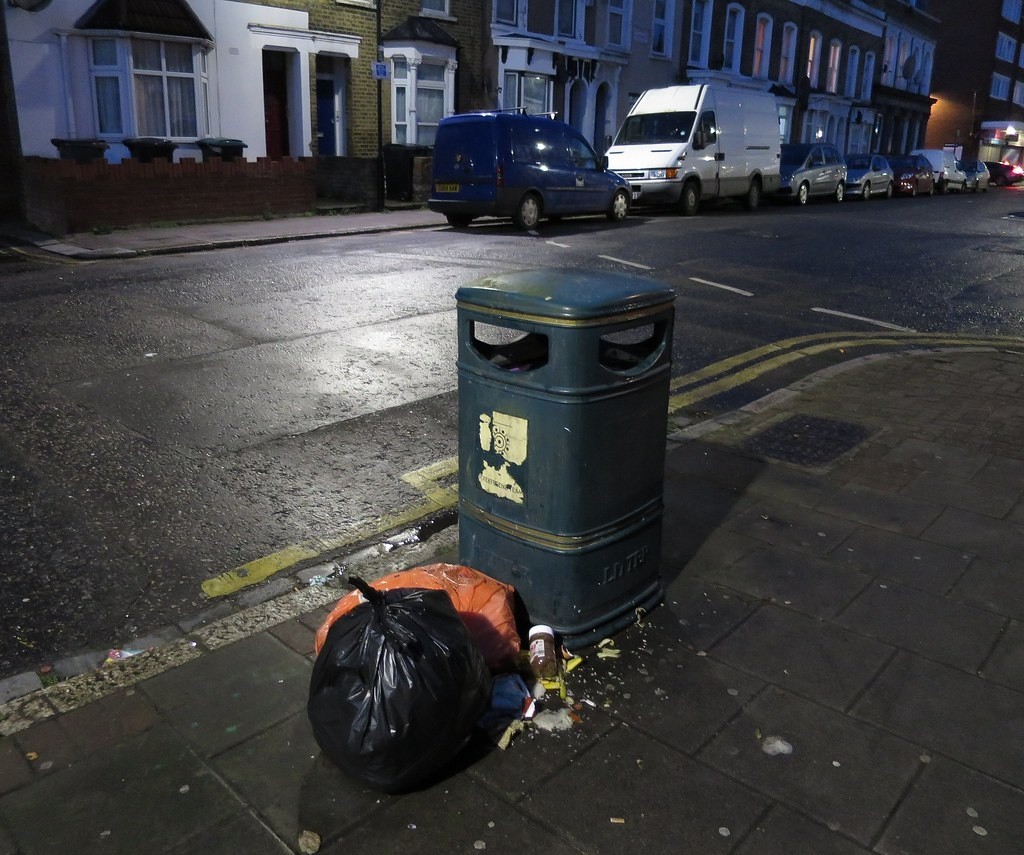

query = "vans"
[605,83,783,218]
[426,108,631,233]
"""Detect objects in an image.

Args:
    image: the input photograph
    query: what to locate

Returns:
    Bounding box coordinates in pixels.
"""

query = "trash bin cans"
[452,266,680,652]
[50,138,110,165]
[195,137,248,162]
[380,143,428,201]
[122,138,179,164]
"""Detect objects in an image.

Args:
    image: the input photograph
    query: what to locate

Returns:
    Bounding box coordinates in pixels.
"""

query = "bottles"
[528,624,556,677]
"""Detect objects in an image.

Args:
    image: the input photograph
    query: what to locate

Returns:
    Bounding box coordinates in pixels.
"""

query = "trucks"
[910,150,967,196]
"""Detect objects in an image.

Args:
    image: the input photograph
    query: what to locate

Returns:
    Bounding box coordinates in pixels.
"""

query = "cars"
[884,153,935,198]
[983,161,1024,187]
[958,160,991,194]
[843,153,894,201]
[759,143,848,209]
